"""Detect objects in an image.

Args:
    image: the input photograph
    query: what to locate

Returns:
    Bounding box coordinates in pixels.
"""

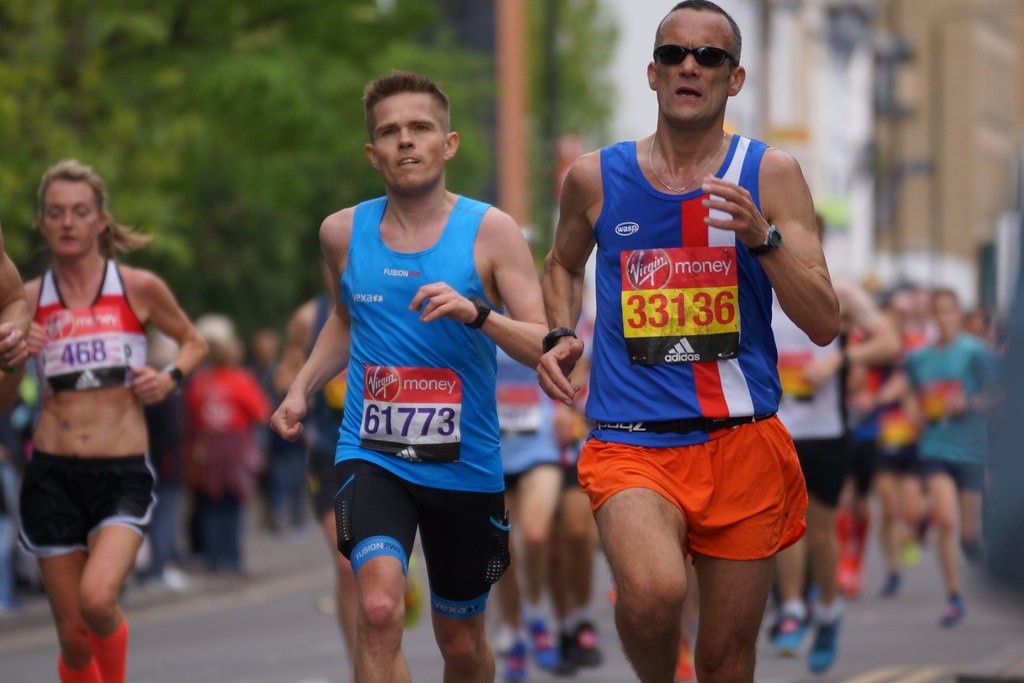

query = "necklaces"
[649,132,727,193]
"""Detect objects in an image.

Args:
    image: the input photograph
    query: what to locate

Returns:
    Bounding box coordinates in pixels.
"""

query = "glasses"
[654,44,738,68]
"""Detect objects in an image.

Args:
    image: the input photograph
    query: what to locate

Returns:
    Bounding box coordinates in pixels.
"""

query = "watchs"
[464,297,491,330]
[748,225,782,258]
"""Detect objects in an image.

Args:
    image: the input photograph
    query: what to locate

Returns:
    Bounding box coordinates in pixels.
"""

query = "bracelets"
[1,368,16,376]
[162,364,184,388]
[543,327,576,353]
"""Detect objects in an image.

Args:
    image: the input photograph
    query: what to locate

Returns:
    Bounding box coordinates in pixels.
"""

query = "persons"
[495,305,602,683]
[0,159,419,683]
[764,209,999,672]
[272,75,550,683]
[538,0,839,683]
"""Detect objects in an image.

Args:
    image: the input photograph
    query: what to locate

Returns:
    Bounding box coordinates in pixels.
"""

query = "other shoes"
[879,574,900,597]
[809,619,839,675]
[496,641,526,682]
[551,635,576,676]
[572,622,601,667]
[525,618,561,671]
[940,597,965,628]
[775,614,807,656]
